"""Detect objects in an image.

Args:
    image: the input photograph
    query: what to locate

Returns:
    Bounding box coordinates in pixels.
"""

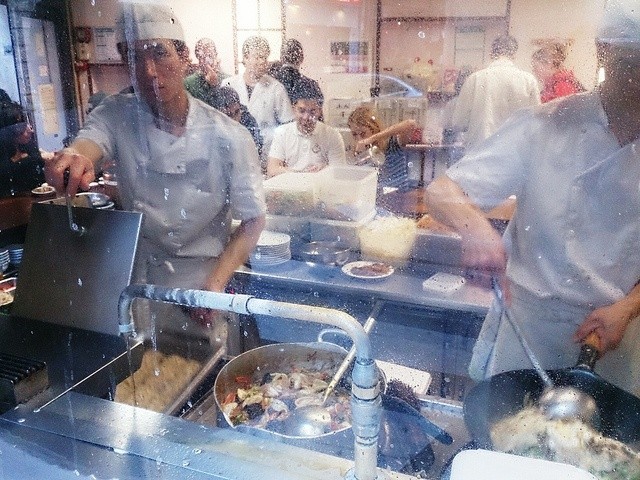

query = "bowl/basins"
[73,191,109,206]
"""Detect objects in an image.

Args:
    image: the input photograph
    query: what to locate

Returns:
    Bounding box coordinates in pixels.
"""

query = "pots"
[301,240,350,270]
[213,327,387,460]
[462,331,639,480]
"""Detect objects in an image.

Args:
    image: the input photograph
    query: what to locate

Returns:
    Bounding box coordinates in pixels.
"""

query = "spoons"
[489,274,601,432]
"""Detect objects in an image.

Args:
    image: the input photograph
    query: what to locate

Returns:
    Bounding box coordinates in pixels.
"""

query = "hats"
[113,1,185,45]
[596,1,639,49]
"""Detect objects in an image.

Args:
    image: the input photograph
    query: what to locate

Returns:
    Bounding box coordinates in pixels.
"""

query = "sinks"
[71,333,231,419]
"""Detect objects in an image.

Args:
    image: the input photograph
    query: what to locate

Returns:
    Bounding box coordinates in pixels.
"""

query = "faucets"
[116,282,247,336]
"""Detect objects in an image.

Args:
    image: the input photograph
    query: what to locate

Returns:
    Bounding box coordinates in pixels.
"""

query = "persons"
[183,37,219,104]
[453,35,540,155]
[266,82,345,176]
[214,86,262,158]
[424,0,640,397]
[347,105,417,191]
[40,1,267,344]
[530,42,588,103]
[219,36,297,172]
[0,103,45,193]
[268,40,324,123]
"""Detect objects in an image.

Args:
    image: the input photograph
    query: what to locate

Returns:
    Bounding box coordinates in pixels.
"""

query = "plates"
[98,180,118,186]
[341,261,394,278]
[243,232,291,267]
[3,243,24,266]
[32,186,55,194]
[0,247,11,271]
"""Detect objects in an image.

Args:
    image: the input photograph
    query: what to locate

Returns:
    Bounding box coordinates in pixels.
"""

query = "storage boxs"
[329,100,356,127]
[313,163,379,222]
[263,171,313,217]
